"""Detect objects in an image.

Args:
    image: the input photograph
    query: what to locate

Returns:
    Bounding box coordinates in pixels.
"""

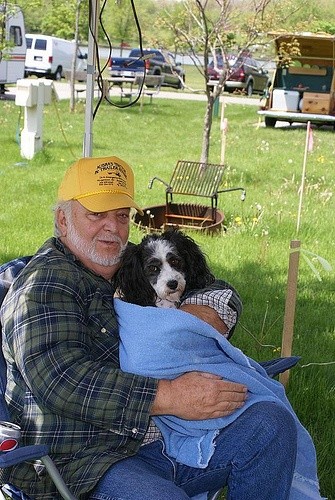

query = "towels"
[112,296,325,500]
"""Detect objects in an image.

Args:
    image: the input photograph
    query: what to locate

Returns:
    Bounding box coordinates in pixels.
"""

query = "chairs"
[0,253,302,500]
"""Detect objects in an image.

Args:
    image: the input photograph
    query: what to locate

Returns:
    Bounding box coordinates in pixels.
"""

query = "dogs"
[113,224,216,311]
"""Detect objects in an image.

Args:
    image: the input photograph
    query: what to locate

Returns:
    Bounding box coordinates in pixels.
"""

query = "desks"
[96,78,137,102]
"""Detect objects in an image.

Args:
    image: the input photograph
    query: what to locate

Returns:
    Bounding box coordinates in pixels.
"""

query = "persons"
[0,156,299,500]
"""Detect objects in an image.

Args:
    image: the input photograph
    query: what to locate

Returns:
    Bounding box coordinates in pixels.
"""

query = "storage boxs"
[272,90,299,111]
[302,90,330,114]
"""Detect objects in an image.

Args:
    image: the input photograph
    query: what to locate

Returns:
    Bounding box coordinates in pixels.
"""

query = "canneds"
[0,421,22,483]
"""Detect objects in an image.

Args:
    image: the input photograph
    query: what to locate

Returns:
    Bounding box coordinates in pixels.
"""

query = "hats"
[58,157,144,217]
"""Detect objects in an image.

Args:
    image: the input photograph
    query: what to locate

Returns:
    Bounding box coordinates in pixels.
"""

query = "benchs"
[66,72,111,101]
[121,75,165,103]
